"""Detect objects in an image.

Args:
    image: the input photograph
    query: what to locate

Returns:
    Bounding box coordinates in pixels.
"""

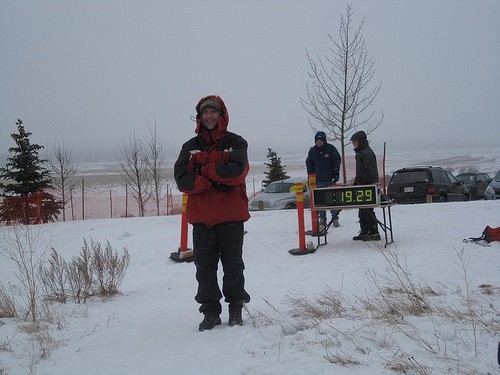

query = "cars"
[248,174,310,211]
[454,171,493,201]
[484,169,500,200]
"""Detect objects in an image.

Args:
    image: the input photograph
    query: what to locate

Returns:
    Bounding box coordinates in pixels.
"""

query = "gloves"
[212,181,233,192]
[193,163,203,175]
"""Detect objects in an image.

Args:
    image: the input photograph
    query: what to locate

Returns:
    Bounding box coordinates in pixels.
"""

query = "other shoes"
[332,217,339,226]
[198,312,221,331]
[228,307,244,326]
[319,217,328,227]
[353,227,381,241]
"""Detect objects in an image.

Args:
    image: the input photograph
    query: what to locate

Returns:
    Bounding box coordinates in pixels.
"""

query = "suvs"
[387,165,472,205]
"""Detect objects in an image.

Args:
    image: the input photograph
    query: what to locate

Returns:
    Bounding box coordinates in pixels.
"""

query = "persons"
[306,131,341,231]
[174,96,249,330]
[350,131,380,241]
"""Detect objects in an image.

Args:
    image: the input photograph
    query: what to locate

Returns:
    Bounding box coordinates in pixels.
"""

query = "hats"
[350,131,367,142]
[314,131,326,143]
[200,100,221,112]
